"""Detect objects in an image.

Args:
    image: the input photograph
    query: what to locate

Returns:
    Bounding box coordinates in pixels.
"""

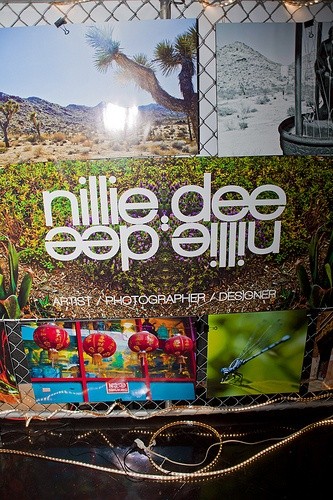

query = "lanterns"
[128,330,159,367]
[163,334,194,365]
[83,332,117,367]
[33,322,70,368]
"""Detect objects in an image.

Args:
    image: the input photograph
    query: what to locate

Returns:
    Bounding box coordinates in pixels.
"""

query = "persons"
[313,25,333,120]
